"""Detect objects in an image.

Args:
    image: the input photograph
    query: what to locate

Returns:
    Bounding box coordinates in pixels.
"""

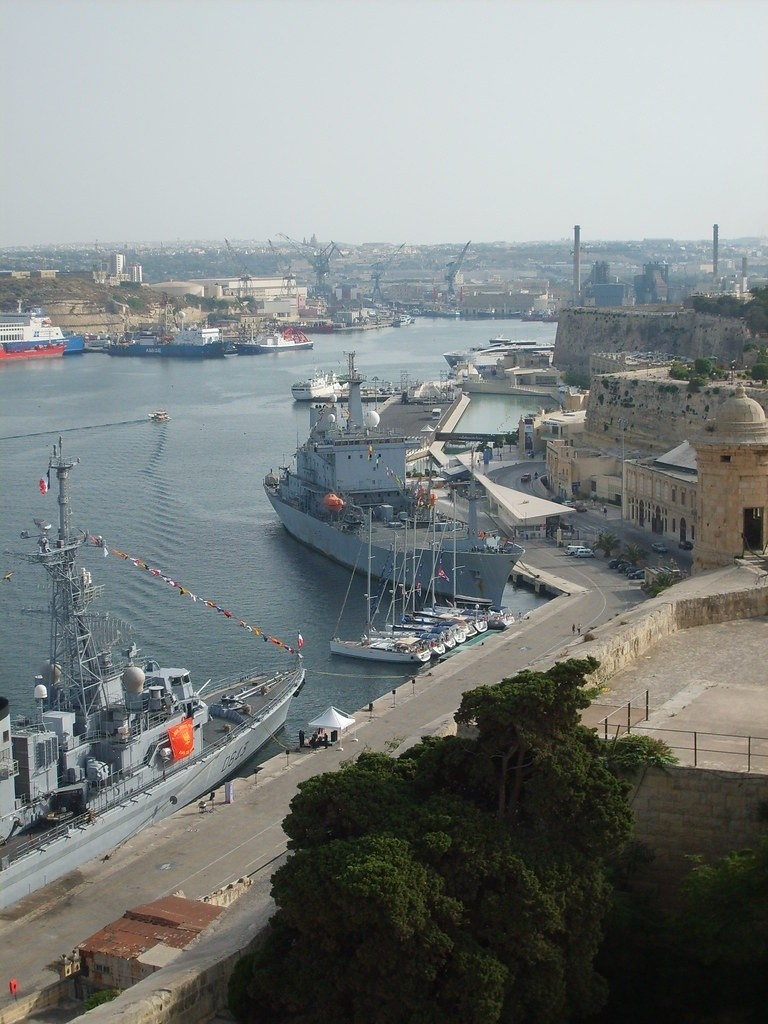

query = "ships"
[262,350,525,613]
[0,434,308,906]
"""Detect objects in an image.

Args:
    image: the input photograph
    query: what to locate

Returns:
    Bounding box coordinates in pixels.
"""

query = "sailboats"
[328,482,516,662]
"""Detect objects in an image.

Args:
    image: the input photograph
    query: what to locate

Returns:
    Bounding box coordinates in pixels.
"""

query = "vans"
[565,546,586,556]
[577,549,596,558]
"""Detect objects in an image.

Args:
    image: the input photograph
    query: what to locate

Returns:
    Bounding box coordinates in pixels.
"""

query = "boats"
[231,321,314,355]
[443,336,555,379]
[107,291,226,358]
[394,312,417,328]
[148,411,172,425]
[291,367,343,401]
[0,297,69,362]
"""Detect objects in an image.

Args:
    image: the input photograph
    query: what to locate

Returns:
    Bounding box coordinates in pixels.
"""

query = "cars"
[561,500,587,514]
[652,542,670,554]
[679,540,693,551]
[608,559,644,579]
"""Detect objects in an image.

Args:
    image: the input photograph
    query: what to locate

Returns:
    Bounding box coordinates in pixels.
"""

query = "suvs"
[520,472,531,482]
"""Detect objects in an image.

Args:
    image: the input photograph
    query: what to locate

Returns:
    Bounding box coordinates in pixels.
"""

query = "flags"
[297,633,304,649]
[440,569,452,582]
[168,716,195,761]
[38,468,50,495]
[365,445,403,488]
[416,582,422,596]
